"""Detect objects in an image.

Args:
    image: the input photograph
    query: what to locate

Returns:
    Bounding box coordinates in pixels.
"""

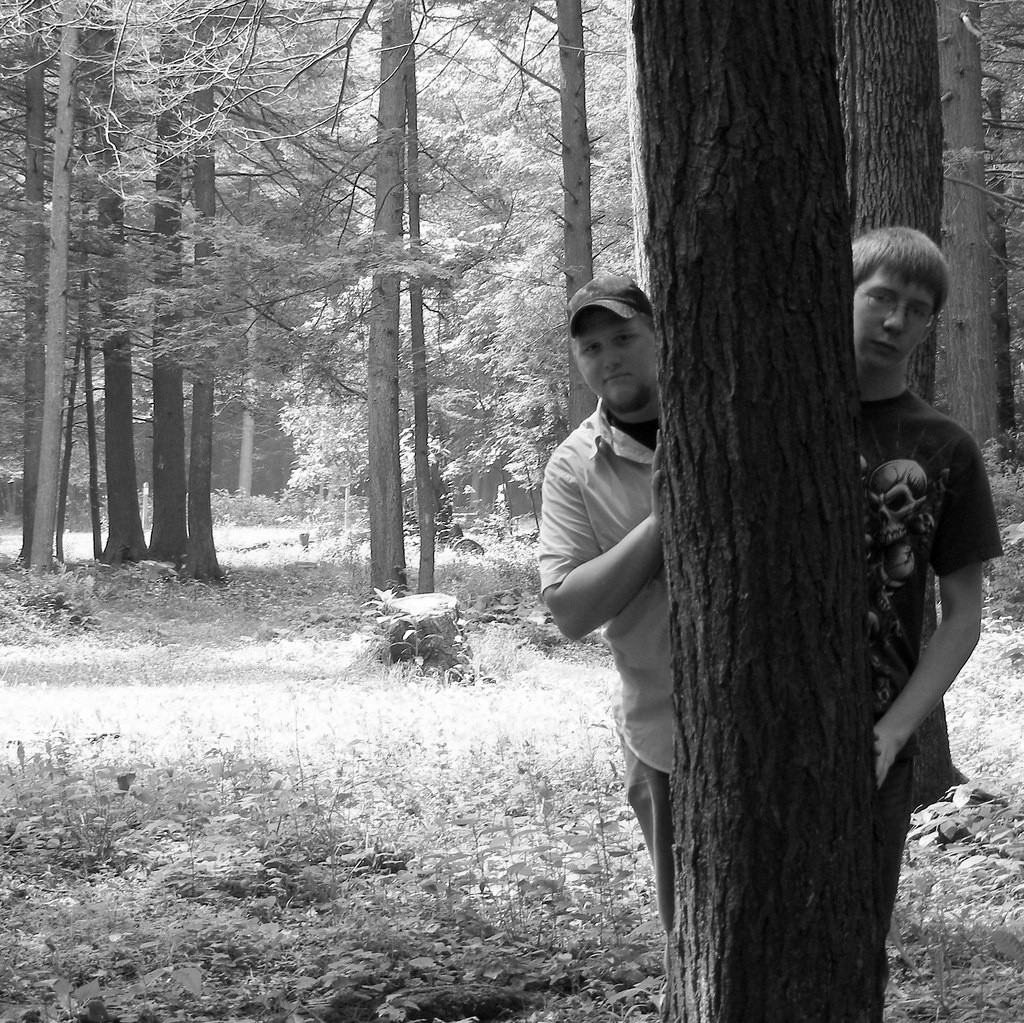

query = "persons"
[539,274,674,973]
[850,228,1003,1023]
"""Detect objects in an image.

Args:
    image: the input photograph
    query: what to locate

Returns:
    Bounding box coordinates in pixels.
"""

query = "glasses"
[855,284,936,329]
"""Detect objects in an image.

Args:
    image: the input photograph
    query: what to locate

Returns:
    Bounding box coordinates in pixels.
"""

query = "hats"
[565,275,652,340]
